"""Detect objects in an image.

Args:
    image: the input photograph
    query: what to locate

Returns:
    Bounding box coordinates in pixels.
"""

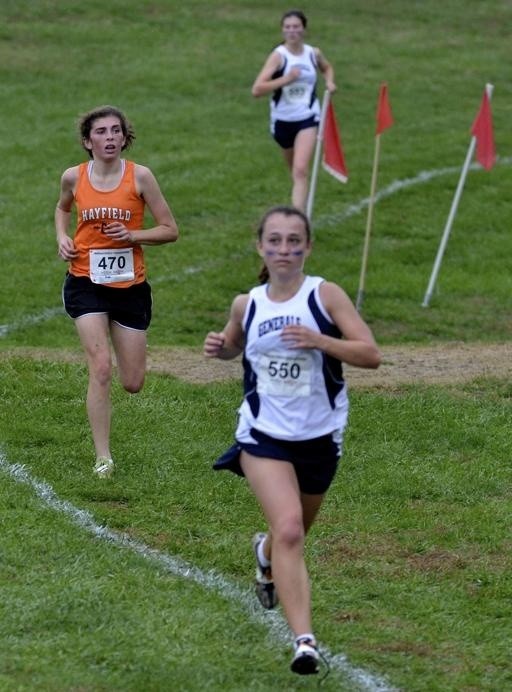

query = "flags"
[469,88,499,172]
[375,79,395,138]
[321,98,349,185]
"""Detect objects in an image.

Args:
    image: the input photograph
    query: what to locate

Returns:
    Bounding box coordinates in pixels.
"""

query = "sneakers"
[289,635,321,675]
[93,456,114,479]
[252,531,280,610]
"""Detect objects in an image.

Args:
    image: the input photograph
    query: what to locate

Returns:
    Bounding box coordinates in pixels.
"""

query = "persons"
[201,202,384,677]
[250,10,339,213]
[52,104,180,480]
